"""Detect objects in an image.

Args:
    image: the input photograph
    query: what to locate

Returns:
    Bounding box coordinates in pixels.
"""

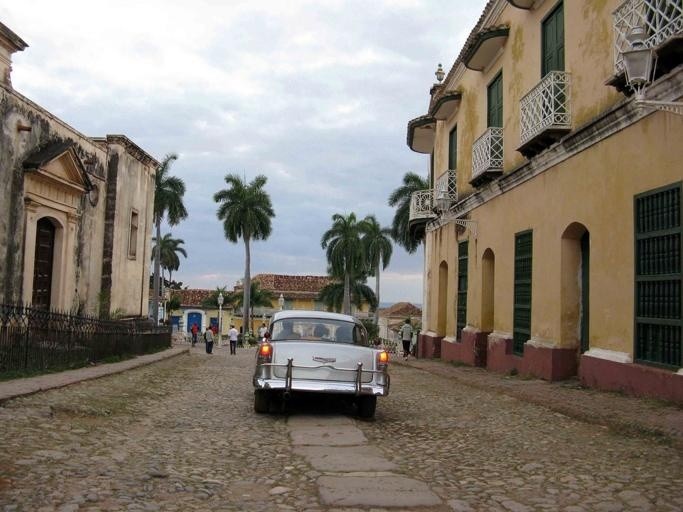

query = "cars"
[251,308,391,423]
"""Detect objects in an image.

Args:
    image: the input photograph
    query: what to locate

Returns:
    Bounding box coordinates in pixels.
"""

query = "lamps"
[615,24,683,119]
[434,186,478,238]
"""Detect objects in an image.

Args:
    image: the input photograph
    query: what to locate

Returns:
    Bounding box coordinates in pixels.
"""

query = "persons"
[398,319,413,362]
[259,323,268,341]
[275,320,300,339]
[301,324,325,340]
[228,325,239,355]
[204,326,214,354]
[190,321,199,347]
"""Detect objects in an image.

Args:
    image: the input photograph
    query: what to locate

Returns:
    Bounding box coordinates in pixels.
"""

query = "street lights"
[277,292,285,310]
[216,292,224,348]
